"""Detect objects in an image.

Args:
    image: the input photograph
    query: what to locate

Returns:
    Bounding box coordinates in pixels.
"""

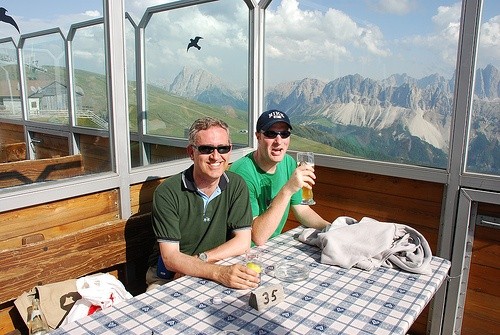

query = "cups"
[296,152,316,205]
[245,248,261,286]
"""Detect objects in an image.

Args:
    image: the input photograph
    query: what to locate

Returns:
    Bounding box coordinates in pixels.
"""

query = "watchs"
[198,252,207,264]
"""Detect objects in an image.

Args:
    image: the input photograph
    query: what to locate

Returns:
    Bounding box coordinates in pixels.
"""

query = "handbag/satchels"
[58,274,134,329]
[12,279,81,332]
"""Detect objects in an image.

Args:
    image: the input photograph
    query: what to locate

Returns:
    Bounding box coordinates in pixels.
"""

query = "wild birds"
[187,37,203,51]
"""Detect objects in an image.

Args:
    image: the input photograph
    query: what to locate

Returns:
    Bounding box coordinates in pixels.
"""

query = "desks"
[45,225,452,335]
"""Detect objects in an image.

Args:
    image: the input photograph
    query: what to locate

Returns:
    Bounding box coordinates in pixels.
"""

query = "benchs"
[0,210,154,305]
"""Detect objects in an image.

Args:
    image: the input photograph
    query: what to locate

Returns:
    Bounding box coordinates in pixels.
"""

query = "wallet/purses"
[156,256,177,279]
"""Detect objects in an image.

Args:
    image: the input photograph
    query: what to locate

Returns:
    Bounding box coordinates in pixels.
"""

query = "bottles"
[29,299,51,335]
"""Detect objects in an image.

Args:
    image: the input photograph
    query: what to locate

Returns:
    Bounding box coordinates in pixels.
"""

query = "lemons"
[246,263,261,274]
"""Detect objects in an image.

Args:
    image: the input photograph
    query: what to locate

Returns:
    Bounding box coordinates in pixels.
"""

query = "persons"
[146,116,261,291]
[228,109,331,248]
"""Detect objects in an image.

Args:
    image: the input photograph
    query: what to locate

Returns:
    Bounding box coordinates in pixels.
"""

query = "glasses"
[191,144,232,154]
[260,129,292,139]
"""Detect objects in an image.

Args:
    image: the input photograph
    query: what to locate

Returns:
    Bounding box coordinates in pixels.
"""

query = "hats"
[256,110,293,132]
